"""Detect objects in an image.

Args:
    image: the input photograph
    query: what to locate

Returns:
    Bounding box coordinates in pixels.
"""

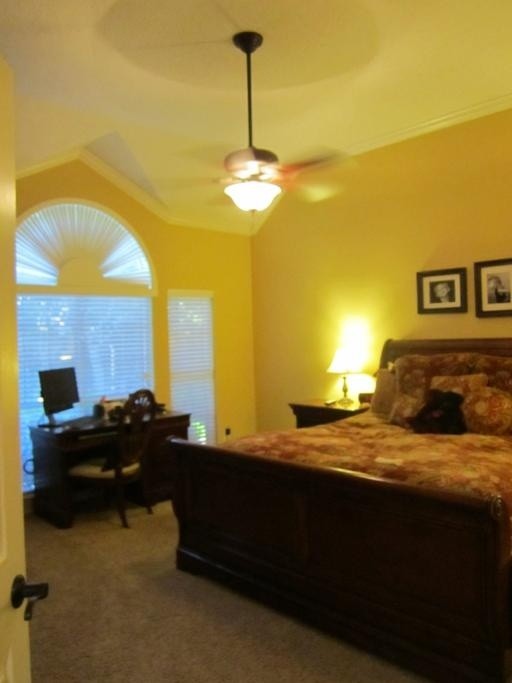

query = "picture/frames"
[416,267,468,314]
[473,257,512,318]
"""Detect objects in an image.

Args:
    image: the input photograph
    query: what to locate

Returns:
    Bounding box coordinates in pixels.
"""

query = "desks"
[28,409,191,529]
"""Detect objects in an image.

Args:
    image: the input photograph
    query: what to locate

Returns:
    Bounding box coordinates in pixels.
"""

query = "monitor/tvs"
[38,367,79,428]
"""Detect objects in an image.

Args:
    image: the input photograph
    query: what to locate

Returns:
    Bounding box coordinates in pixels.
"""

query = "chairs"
[68,388,156,529]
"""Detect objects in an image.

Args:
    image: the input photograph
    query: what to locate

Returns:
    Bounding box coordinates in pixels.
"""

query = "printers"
[94,398,126,420]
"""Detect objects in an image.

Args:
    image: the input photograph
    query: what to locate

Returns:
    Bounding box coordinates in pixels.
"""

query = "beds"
[166,337,512,683]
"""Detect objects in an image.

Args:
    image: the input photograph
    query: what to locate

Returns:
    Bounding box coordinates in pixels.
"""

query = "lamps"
[326,352,363,404]
[225,183,280,212]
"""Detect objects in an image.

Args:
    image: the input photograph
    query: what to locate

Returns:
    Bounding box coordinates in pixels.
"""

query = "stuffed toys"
[406,389,468,433]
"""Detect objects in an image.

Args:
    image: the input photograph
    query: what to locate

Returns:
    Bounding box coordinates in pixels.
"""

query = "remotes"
[325,400,336,405]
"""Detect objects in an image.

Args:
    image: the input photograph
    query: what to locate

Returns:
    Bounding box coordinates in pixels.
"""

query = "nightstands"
[289,399,369,428]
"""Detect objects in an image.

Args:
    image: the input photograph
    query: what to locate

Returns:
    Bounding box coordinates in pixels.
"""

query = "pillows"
[370,352,512,435]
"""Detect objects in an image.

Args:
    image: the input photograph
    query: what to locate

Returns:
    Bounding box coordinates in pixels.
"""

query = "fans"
[211,31,342,203]
